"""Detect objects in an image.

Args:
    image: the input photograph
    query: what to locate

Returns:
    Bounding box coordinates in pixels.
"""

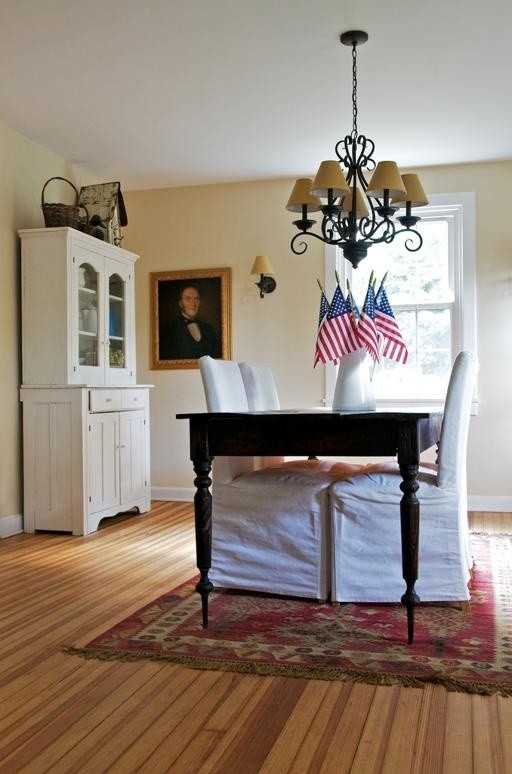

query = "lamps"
[284,30,429,270]
[249,254,276,301]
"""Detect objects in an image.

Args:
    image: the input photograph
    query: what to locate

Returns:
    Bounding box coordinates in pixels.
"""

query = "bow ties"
[186,319,201,325]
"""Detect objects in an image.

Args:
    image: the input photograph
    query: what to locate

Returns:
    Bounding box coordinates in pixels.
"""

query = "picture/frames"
[147,265,233,371]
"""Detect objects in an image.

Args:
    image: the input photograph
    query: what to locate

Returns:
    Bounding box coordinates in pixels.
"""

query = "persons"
[160,285,219,361]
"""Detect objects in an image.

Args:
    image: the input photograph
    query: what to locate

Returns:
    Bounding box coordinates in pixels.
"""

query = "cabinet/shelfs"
[18,226,154,536]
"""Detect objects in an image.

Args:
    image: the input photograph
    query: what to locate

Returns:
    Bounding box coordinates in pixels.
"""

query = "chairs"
[329,350,475,612]
[199,355,331,605]
[239,361,366,477]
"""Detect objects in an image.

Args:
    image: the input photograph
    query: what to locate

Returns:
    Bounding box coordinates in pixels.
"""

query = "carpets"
[59,529,512,699]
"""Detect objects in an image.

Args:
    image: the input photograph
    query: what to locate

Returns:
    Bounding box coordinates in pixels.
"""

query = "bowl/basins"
[79,358,86,365]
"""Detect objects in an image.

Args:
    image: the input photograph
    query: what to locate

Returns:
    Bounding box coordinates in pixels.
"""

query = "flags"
[311,277,411,369]
